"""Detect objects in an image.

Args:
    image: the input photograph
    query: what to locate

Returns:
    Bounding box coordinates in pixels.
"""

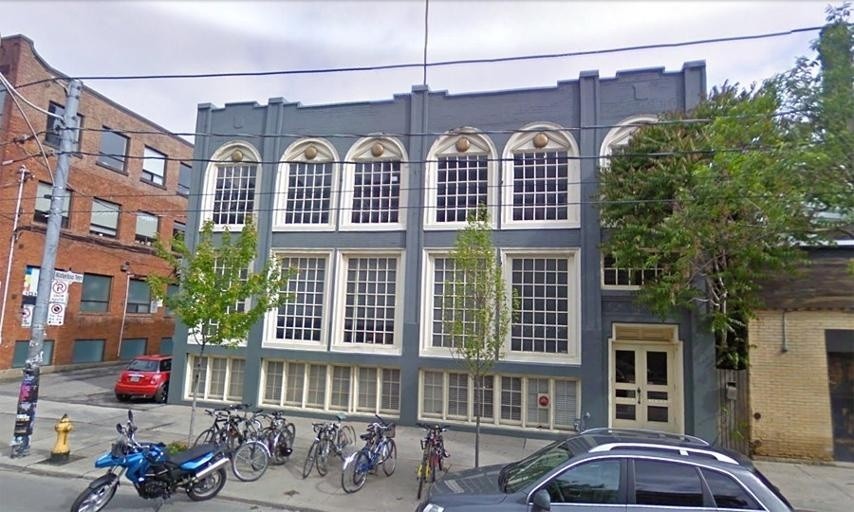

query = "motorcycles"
[71,411,230,512]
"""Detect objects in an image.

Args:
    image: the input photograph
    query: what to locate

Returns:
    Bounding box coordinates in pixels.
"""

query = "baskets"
[381,423,396,439]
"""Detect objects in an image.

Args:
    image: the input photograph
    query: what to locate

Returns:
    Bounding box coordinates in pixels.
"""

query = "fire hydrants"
[50,412,72,460]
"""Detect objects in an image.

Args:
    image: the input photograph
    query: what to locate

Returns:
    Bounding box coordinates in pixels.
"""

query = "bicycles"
[303,413,398,494]
[415,422,451,499]
[193,404,295,482]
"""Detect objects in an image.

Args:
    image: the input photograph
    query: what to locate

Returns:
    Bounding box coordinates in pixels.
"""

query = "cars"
[114,354,171,403]
[417,426,794,512]
